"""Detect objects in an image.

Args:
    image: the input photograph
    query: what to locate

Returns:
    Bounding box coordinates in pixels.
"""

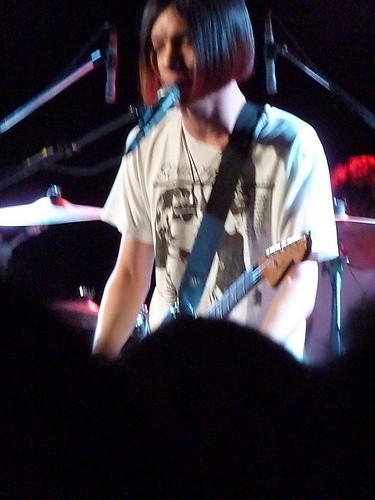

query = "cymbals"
[334,215,375,225]
[0,198,102,226]
[53,300,99,315]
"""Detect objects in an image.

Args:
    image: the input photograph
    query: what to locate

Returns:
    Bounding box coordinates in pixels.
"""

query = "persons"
[89,0,337,367]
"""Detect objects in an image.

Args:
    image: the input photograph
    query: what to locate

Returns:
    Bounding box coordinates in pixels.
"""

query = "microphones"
[264,7,278,96]
[105,29,117,105]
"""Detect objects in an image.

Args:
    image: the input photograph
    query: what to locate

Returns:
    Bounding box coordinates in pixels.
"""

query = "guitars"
[195,232,312,318]
